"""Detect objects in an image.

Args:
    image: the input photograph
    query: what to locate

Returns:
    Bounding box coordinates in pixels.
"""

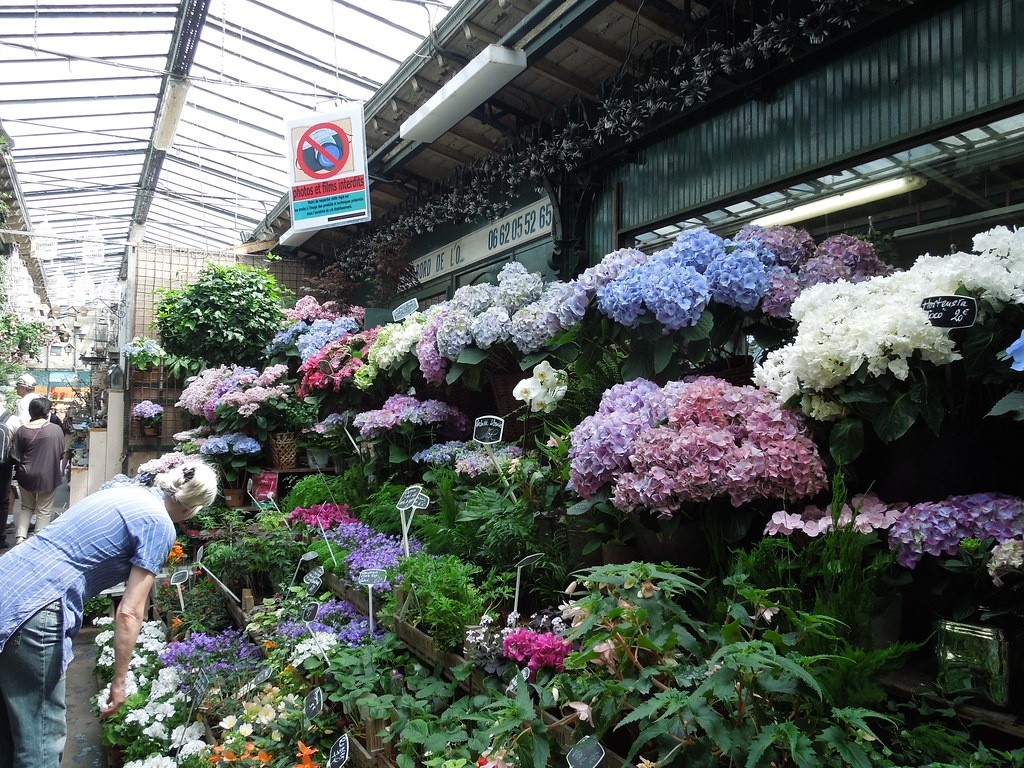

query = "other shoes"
[60,473,65,476]
[0,537,9,549]
[16,537,25,546]
[6,521,17,534]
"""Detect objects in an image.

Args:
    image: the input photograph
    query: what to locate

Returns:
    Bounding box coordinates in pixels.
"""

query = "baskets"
[260,431,299,469]
[489,370,544,446]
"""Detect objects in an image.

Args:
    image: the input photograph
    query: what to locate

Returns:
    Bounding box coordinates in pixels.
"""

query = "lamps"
[129,221,148,244]
[748,173,929,229]
[400,43,527,144]
[152,75,191,151]
[279,228,319,247]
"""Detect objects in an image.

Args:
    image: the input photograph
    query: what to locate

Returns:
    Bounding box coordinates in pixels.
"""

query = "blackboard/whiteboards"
[359,569,388,586]
[307,449,318,465]
[411,493,429,509]
[473,415,504,445]
[171,570,188,584]
[303,566,323,581]
[396,486,421,509]
[191,668,208,704]
[304,687,323,721]
[253,666,272,685]
[330,733,349,767]
[302,603,319,623]
[921,295,977,329]
[302,551,318,561]
[306,577,322,595]
[566,735,605,768]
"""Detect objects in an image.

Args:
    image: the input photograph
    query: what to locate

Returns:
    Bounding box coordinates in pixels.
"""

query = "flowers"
[89,224,1024,768]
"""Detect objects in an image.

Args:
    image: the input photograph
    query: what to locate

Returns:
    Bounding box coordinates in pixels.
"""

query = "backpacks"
[0,409,14,463]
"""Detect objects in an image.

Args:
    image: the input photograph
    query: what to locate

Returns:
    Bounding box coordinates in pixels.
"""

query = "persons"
[0,374,71,550]
[0,461,217,768]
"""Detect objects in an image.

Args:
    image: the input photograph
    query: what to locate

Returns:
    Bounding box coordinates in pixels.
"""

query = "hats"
[9,374,36,389]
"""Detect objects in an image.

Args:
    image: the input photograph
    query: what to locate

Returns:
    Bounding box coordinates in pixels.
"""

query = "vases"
[144,428,156,435]
[307,448,329,470]
[600,539,662,585]
[223,489,246,507]
[935,616,1024,711]
[534,520,554,539]
[567,516,602,564]
[818,588,903,648]
